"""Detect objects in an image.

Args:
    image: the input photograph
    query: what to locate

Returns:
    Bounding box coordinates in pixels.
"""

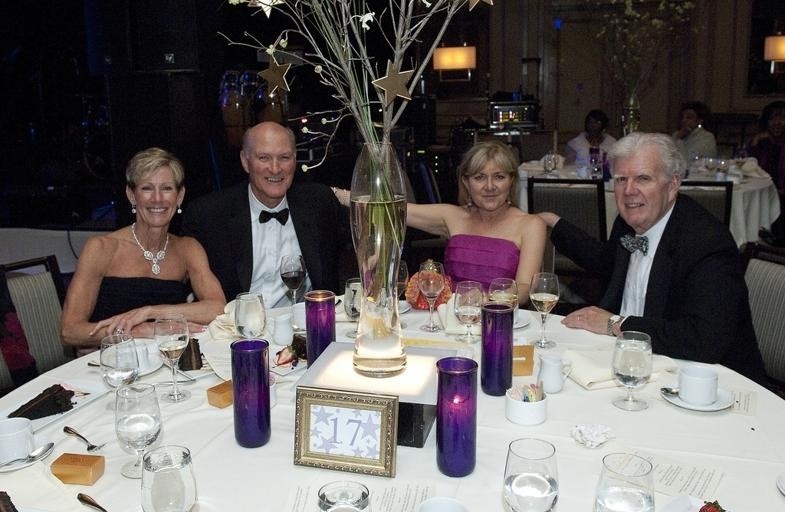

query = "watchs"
[606,314,626,338]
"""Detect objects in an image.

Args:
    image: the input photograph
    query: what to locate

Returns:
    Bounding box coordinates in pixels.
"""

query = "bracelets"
[331,185,338,193]
[342,189,348,207]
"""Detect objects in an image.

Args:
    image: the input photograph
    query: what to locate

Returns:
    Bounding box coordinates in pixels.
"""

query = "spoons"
[0,442,54,467]
[661,388,679,396]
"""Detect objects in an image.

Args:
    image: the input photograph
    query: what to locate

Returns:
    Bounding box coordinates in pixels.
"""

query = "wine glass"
[529,272,560,350]
[279,254,306,332]
[454,281,485,344]
[154,313,192,403]
[397,260,409,330]
[344,277,362,339]
[612,330,652,411]
[586,143,603,179]
[235,291,275,386]
[488,277,519,341]
[544,154,560,179]
[100,334,139,412]
[115,383,162,478]
[417,261,445,332]
[692,152,748,191]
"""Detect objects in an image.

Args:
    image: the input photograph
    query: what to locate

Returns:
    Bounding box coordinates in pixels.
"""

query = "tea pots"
[537,352,574,393]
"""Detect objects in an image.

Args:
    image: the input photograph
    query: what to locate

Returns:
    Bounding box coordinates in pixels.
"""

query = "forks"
[63,426,107,453]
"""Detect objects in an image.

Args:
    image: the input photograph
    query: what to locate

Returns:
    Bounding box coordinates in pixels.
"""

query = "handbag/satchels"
[405,258,452,310]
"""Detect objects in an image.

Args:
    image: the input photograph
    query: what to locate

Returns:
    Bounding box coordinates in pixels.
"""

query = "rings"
[114,327,125,334]
[577,315,581,321]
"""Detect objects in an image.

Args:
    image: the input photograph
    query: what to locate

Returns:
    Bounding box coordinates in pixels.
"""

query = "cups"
[502,437,560,511]
[318,480,370,512]
[481,303,514,395]
[303,289,335,369]
[137,445,199,512]
[0,417,33,464]
[229,338,271,448]
[594,453,655,512]
[436,356,478,477]
[678,365,719,406]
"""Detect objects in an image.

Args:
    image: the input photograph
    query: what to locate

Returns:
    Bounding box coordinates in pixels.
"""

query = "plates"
[514,316,530,329]
[397,299,411,315]
[660,387,736,412]
[0,449,53,473]
[100,338,164,377]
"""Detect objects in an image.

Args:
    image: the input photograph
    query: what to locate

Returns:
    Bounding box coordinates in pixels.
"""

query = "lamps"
[431,40,478,85]
[764,31,785,76]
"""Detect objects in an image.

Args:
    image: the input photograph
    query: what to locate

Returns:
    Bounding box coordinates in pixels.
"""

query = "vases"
[622,85,646,139]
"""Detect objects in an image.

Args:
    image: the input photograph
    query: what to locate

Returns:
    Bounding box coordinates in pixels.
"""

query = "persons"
[563,108,617,162]
[667,99,717,160]
[535,132,769,387]
[328,139,550,308]
[178,122,356,309]
[744,101,784,197]
[59,142,229,357]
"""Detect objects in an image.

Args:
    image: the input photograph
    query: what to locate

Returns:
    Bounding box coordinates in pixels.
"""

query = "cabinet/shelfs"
[425,96,489,155]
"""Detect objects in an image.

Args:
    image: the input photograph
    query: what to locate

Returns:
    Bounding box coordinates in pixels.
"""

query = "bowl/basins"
[506,387,548,424]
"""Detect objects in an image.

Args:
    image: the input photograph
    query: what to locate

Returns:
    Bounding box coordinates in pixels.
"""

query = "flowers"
[590,1,716,137]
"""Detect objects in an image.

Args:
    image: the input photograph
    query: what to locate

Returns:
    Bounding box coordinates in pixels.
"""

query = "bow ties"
[259,207,289,226]
[619,234,649,256]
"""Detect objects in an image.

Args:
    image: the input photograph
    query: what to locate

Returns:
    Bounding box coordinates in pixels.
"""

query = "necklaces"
[585,135,604,144]
[132,222,172,277]
